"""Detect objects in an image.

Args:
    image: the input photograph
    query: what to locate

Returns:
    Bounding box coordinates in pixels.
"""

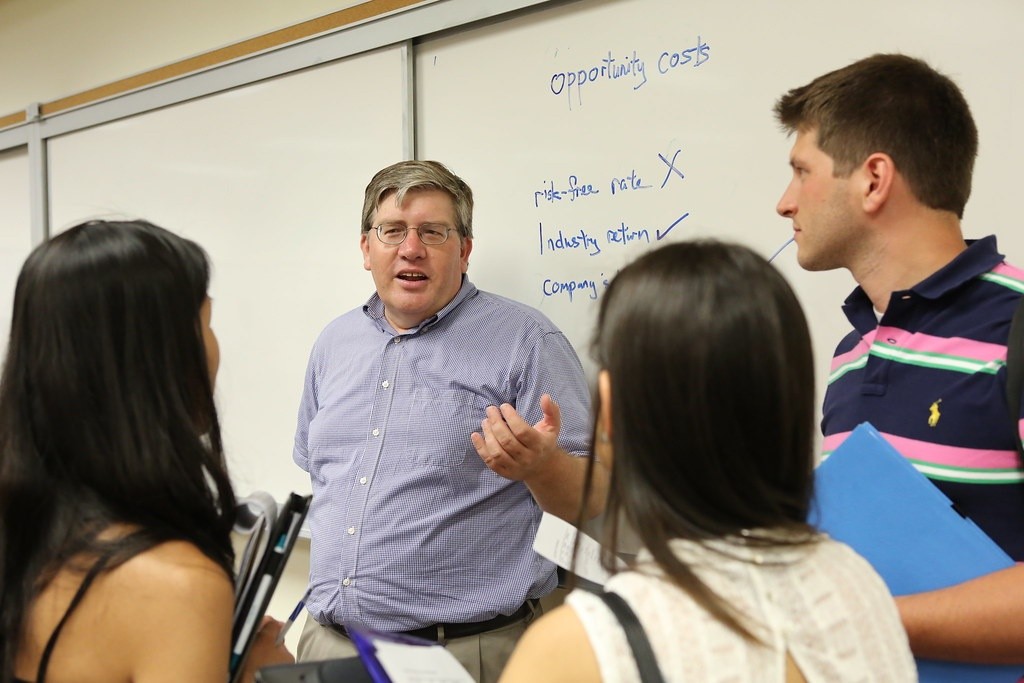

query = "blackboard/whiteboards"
[0,0,1021,465]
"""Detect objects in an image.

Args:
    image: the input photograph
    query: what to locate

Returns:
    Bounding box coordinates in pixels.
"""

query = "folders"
[233,493,315,675]
[808,419,1024,683]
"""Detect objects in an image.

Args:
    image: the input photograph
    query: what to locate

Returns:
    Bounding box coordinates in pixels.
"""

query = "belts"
[316,598,536,645]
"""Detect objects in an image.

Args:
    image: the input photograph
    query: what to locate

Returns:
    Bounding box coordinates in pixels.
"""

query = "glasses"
[367,222,460,246]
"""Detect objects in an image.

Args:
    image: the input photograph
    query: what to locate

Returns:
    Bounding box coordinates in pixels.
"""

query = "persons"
[773,51,1024,682]
[0,219,296,683]
[497,236,917,681]
[291,159,608,683]
